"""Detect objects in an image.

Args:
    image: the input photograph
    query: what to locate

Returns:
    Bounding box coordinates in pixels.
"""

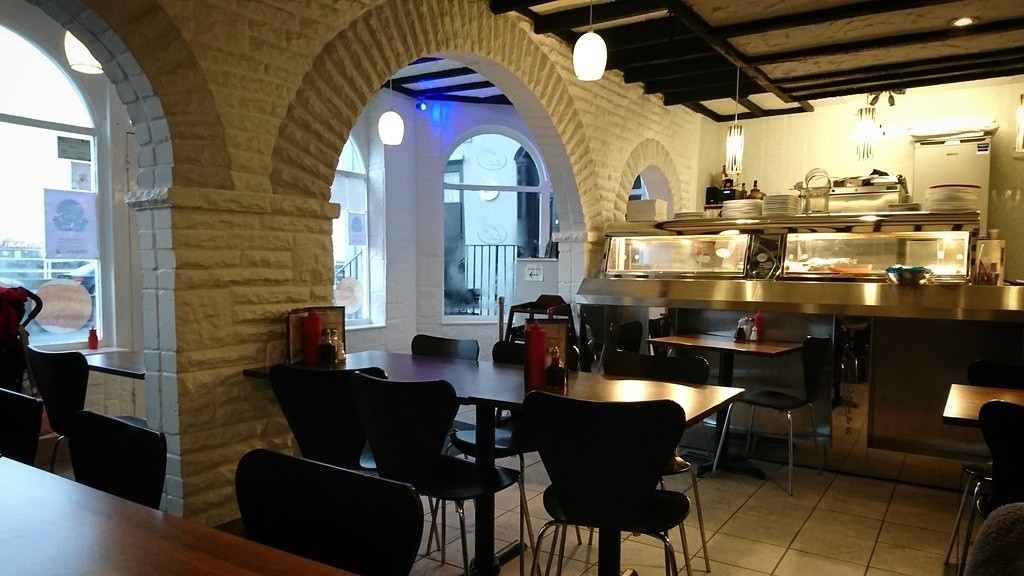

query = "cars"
[56,263,96,296]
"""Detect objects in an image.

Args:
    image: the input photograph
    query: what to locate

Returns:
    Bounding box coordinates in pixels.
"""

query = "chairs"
[1,333,1024,576]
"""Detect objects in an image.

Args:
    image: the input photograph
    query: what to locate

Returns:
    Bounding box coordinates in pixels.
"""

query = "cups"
[988,229,1000,240]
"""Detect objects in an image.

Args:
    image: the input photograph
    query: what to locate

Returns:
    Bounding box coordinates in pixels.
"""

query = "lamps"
[856,93,878,159]
[715,237,736,258]
[725,68,744,175]
[573,0,607,81]
[377,77,405,146]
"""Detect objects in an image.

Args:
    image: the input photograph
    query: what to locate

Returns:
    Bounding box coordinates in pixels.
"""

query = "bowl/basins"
[887,273,931,285]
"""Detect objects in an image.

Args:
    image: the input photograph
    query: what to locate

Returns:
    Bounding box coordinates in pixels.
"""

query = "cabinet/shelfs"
[0,258,99,324]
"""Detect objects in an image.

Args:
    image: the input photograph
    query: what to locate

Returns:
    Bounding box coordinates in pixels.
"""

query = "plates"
[763,196,800,216]
[927,185,982,211]
[722,200,762,217]
[676,212,707,219]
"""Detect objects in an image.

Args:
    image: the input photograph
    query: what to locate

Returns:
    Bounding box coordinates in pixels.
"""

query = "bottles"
[750,180,760,194]
[526,320,546,388]
[722,165,728,188]
[88,326,98,349]
[547,347,565,389]
[305,311,321,364]
[798,190,807,213]
[734,317,754,340]
[755,311,763,340]
[740,183,747,199]
[750,326,758,341]
[317,329,346,364]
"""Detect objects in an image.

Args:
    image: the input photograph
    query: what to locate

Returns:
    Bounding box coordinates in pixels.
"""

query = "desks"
[80,349,146,380]
[1,457,350,575]
[243,350,745,576]
[646,331,806,477]
[941,384,1024,425]
